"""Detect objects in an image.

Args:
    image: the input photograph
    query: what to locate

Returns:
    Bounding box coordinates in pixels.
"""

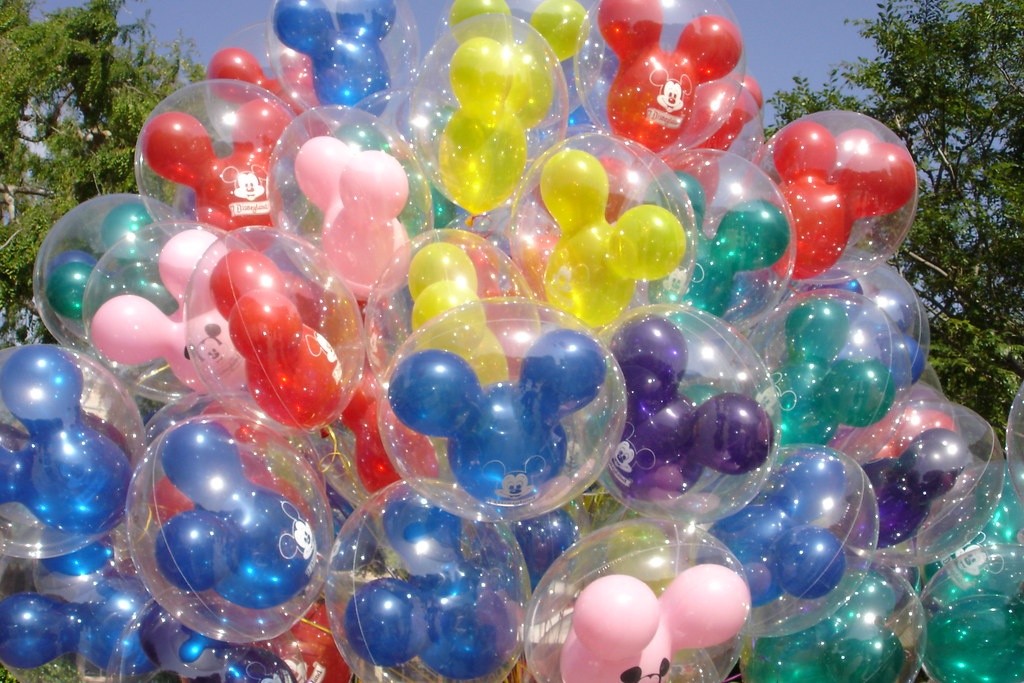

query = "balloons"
[0,2,1024,683]
[742,561,927,682]
[524,520,754,683]
[324,477,532,683]
[126,413,334,644]
[917,545,1024,683]
[699,445,880,636]
[107,582,306,683]
